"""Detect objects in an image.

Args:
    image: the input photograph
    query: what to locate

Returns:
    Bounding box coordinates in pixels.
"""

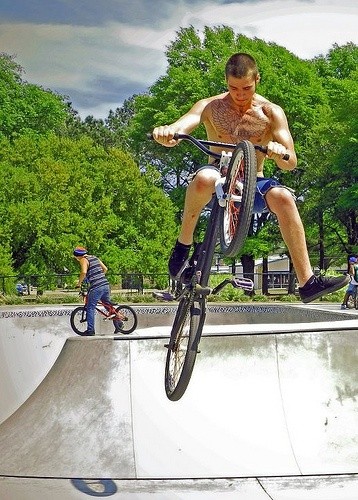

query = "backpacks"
[354,264,358,283]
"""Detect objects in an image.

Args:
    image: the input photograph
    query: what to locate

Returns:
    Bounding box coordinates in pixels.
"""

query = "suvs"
[122,273,143,289]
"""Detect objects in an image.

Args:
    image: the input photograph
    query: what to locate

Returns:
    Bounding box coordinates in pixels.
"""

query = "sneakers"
[298,272,349,303]
[169,239,191,278]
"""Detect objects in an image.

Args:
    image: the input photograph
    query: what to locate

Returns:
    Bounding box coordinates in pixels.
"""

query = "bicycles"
[145,129,290,401]
[70,285,138,337]
[344,271,358,310]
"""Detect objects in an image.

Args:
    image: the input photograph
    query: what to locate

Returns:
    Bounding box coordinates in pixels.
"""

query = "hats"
[73,247,88,256]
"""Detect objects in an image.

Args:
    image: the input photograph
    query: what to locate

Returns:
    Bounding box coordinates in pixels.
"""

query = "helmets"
[349,257,358,263]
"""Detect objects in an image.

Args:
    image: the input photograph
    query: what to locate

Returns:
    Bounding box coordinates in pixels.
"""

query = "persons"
[153,53,350,304]
[341,257,358,310]
[73,245,124,336]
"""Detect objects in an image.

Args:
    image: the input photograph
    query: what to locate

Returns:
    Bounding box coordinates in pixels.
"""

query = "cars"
[16,284,26,295]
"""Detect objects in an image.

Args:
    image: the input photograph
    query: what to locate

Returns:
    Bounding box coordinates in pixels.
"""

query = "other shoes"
[81,330,95,336]
[114,322,125,334]
[341,304,346,310]
